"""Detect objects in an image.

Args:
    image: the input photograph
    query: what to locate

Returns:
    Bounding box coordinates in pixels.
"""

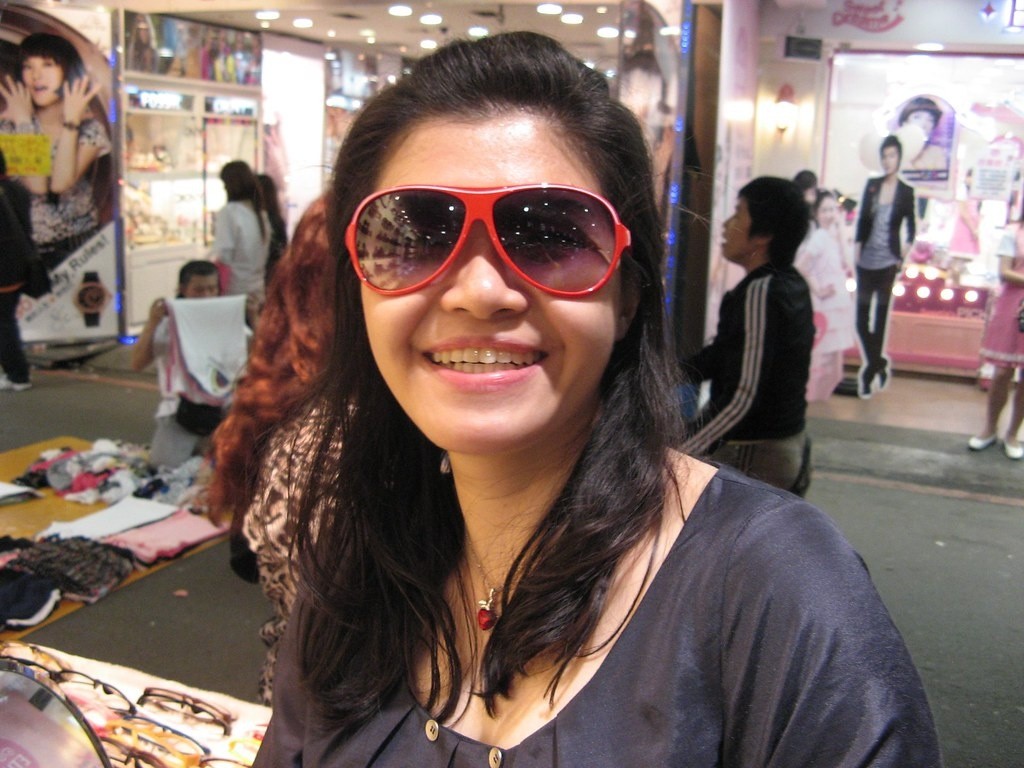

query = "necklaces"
[466,538,521,634]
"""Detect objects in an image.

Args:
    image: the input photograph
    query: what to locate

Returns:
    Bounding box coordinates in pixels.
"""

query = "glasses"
[343,185,631,298]
[0,639,254,768]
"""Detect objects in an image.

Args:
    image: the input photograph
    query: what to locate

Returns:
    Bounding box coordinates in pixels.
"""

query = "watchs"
[72,271,111,330]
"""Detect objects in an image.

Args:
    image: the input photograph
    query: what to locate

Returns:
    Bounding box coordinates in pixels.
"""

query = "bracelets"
[62,122,81,132]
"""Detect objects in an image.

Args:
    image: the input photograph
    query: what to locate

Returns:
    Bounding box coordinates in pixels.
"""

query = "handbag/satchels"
[213,257,230,297]
[1019,308,1024,331]
[176,395,224,437]
[23,258,48,298]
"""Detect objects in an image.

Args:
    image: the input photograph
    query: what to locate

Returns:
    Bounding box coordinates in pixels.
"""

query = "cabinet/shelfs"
[841,311,985,380]
[125,81,265,335]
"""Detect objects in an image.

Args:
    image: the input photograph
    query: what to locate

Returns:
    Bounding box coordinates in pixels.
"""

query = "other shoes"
[0,374,32,392]
[1003,438,1023,459]
[0,572,61,631]
[969,431,997,450]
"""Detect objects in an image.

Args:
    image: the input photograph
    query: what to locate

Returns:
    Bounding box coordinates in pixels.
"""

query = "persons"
[200,189,367,706]
[967,201,1024,459]
[791,98,982,396]
[0,149,40,392]
[252,31,941,768]
[0,34,110,267]
[131,158,290,466]
[673,175,813,497]
